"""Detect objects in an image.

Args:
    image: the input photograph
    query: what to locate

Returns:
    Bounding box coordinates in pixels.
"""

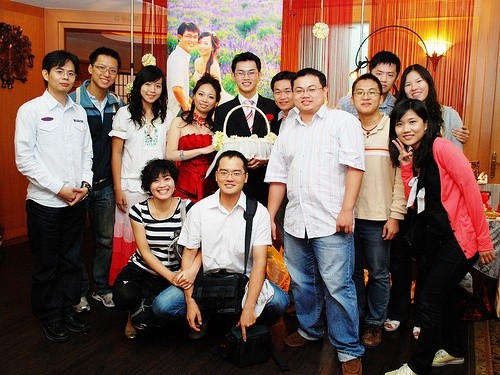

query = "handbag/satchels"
[201,271,249,330]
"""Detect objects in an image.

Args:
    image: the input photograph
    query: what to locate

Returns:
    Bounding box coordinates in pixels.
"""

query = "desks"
[471,217,500,318]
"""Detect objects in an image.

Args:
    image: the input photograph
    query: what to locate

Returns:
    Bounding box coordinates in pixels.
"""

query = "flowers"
[212,131,277,151]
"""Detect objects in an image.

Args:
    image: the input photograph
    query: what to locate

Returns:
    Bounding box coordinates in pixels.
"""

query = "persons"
[152,150,289,342]
[67,47,126,313]
[263,68,365,375]
[337,50,469,144]
[350,73,408,348]
[166,22,235,132]
[108,65,173,338]
[213,51,283,208]
[385,99,496,375]
[164,76,221,204]
[111,158,202,338]
[269,70,297,316]
[384,63,464,341]
[14,50,93,342]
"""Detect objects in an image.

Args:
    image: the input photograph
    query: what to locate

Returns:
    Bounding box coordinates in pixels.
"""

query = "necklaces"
[359,119,378,138]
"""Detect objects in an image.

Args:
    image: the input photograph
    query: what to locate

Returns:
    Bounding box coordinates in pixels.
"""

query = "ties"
[243,101,255,134]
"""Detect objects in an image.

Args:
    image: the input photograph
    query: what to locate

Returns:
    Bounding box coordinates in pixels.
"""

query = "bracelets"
[179,150,184,161]
[80,183,92,201]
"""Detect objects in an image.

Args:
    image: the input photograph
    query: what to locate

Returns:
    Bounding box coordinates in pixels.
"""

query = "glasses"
[234,72,258,77]
[354,88,381,97]
[294,86,323,94]
[92,63,118,75]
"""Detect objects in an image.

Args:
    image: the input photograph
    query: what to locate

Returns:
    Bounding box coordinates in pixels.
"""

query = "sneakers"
[432,349,464,366]
[384,363,417,375]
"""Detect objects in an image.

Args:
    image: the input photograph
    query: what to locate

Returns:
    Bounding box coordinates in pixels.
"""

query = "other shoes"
[284,331,307,347]
[66,314,90,333]
[41,319,70,341]
[412,326,420,339]
[363,326,381,347]
[383,319,399,333]
[76,297,91,313]
[341,356,362,374]
[92,292,115,308]
[125,319,136,339]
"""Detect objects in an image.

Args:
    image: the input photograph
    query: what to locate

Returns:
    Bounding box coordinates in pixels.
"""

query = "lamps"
[312,0,330,39]
[142,0,157,67]
[418,40,455,71]
